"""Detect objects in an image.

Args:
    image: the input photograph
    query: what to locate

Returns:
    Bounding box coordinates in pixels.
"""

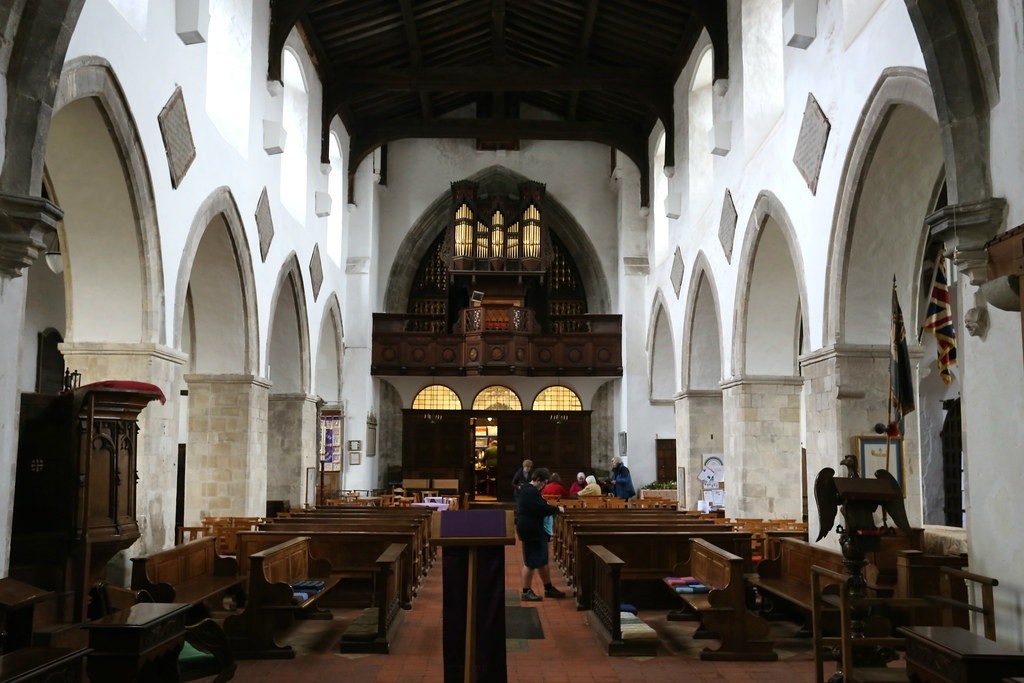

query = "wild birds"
[814,454,913,542]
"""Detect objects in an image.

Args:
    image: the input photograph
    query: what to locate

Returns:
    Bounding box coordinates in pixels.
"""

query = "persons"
[541,472,568,499]
[512,460,533,525]
[482,440,497,472]
[514,467,565,601]
[605,457,635,507]
[568,472,602,497]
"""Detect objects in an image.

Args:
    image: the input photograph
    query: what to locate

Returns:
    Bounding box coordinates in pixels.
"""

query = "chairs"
[326,487,470,511]
[542,495,679,509]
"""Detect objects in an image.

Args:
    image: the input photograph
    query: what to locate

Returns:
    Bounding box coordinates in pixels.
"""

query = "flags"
[918,248,958,384]
[888,289,916,444]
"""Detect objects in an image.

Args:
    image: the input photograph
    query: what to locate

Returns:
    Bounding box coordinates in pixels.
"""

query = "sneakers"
[545,587,565,598]
[521,590,542,601]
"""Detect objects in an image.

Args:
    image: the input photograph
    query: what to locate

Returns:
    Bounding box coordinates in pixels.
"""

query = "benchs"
[130,505,437,683]
[553,508,845,662]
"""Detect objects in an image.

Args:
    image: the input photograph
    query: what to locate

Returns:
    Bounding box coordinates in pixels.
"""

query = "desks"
[402,479,430,489]
[432,479,459,493]
[80,602,193,683]
[0,646,94,683]
[896,626,1024,683]
